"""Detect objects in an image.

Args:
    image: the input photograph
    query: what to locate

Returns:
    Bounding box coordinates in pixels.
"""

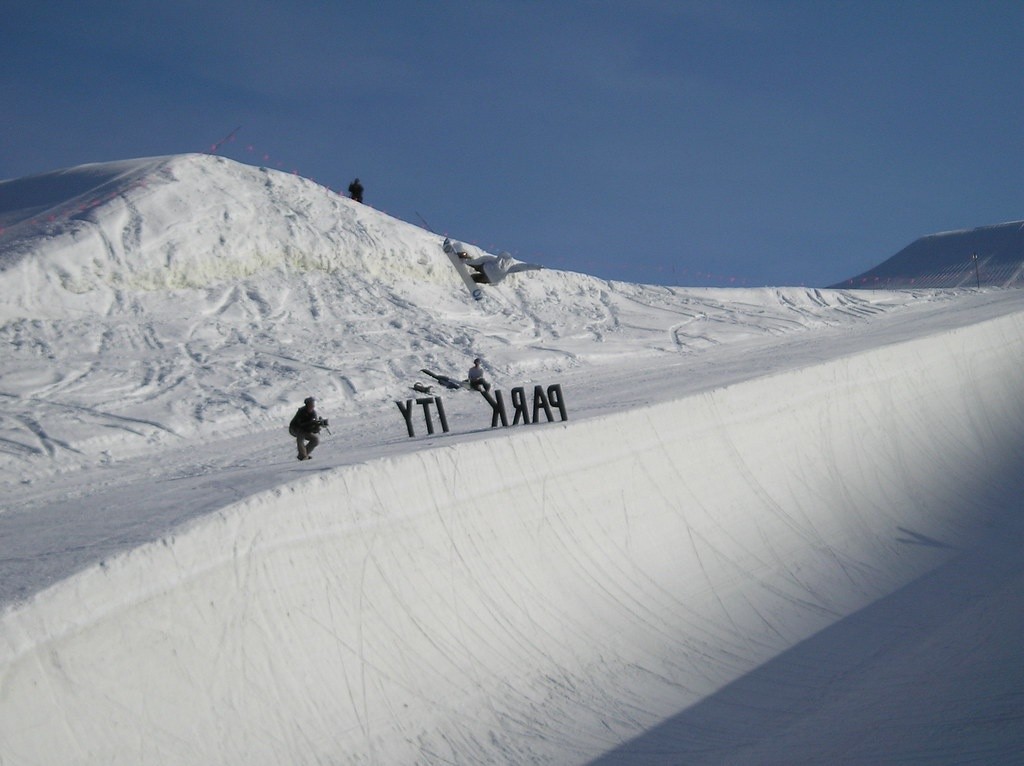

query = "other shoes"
[297,456,312,461]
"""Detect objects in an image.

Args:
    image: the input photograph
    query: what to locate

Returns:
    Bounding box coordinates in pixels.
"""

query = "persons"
[348,178,364,204]
[468,358,491,392]
[289,397,321,461]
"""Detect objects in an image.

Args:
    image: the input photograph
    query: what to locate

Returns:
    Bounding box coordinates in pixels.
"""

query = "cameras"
[311,417,328,433]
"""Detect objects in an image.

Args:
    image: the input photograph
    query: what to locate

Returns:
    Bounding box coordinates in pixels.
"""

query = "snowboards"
[442,238,484,301]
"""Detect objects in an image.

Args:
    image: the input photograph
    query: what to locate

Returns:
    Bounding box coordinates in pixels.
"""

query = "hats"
[474,358,481,364]
